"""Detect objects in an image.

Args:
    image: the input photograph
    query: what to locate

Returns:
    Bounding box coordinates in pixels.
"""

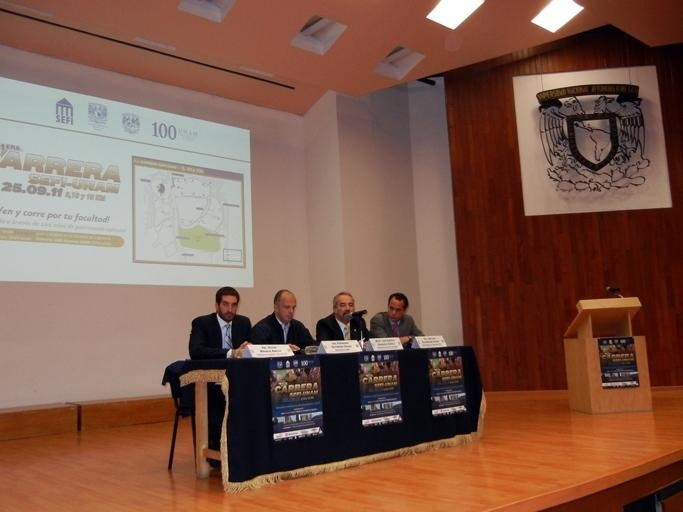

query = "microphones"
[606,287,620,292]
[349,310,367,318]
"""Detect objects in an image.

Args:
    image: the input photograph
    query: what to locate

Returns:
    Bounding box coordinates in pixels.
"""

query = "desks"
[183,344,471,478]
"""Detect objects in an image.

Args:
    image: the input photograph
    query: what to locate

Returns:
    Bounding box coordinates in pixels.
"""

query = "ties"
[344,327,349,340]
[224,324,231,349]
[392,321,398,336]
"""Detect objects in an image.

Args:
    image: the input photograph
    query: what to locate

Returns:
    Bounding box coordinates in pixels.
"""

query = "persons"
[316,292,374,346]
[189,287,253,360]
[251,290,317,351]
[370,293,425,346]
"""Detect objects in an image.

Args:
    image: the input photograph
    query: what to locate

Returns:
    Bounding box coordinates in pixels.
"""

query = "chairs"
[167,361,195,470]
